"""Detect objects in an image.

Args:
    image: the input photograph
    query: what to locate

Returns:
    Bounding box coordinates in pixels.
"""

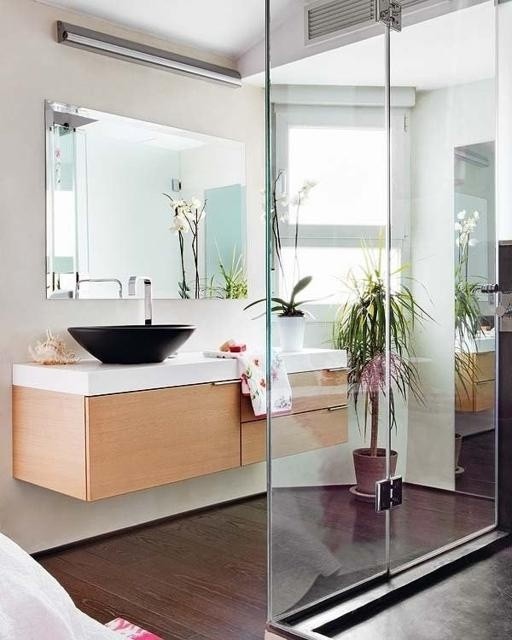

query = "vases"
[277,316,305,352]
[456,435,464,473]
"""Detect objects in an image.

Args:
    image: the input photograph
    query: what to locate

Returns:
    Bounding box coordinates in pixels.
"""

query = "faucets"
[76,278,123,299]
[127,275,152,325]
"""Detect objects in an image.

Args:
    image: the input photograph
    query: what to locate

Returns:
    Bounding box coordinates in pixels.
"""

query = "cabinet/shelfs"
[13,353,347,501]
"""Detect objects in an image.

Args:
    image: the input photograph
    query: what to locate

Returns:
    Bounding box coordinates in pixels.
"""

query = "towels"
[204,352,294,417]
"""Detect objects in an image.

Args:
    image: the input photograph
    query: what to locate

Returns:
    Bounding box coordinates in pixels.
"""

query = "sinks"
[67,325,197,364]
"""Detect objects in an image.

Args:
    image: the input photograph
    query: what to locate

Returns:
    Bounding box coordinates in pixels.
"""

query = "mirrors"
[453,140,498,500]
[43,102,247,302]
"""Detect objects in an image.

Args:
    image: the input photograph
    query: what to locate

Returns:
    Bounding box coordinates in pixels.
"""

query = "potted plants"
[336,226,437,503]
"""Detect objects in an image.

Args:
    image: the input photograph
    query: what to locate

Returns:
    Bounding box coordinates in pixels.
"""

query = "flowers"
[455,209,487,412]
[243,168,334,319]
[162,192,208,302]
